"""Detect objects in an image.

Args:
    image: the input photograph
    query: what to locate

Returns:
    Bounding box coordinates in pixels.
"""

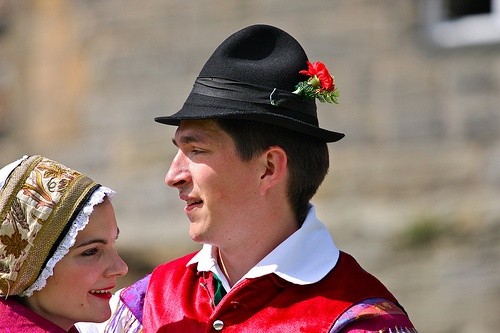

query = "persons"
[77,24,417,333]
[1,156,129,333]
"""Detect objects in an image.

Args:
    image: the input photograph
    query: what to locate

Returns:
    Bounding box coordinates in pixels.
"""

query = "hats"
[152,24,345,142]
[0,154,117,297]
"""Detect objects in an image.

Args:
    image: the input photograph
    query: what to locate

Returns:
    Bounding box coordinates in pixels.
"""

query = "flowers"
[268,59,339,111]
